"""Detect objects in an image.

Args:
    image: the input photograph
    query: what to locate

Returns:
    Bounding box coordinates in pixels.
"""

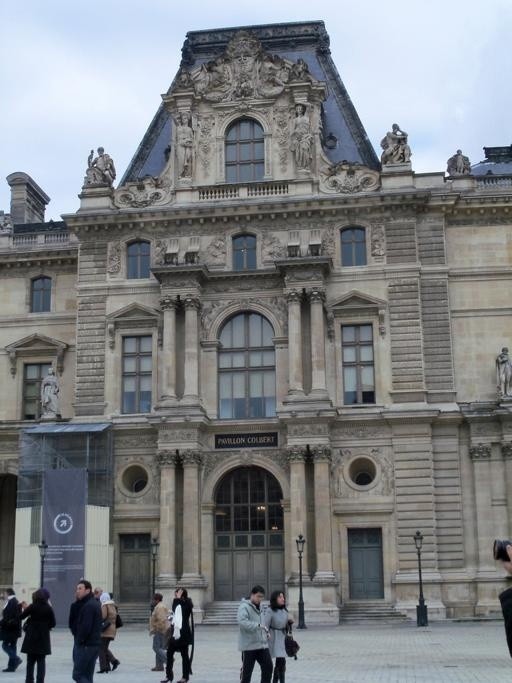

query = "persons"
[148,588,194,683]
[264,590,295,683]
[40,367,59,415]
[0,587,56,683]
[86,147,116,185]
[495,347,512,396]
[447,150,471,176]
[380,124,411,164]
[499,544,512,662]
[68,580,120,683]
[175,113,194,179]
[287,104,313,169]
[236,585,273,683]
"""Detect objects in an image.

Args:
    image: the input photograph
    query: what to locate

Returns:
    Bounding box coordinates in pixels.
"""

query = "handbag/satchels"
[161,624,174,649]
[285,636,299,657]
[116,615,122,628]
[100,619,111,633]
[23,617,32,632]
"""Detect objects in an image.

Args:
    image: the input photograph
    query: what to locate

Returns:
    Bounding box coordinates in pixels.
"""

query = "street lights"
[294,535,311,631]
[412,529,431,628]
[36,538,51,588]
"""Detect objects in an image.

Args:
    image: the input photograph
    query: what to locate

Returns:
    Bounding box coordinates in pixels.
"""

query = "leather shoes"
[2,658,189,682]
